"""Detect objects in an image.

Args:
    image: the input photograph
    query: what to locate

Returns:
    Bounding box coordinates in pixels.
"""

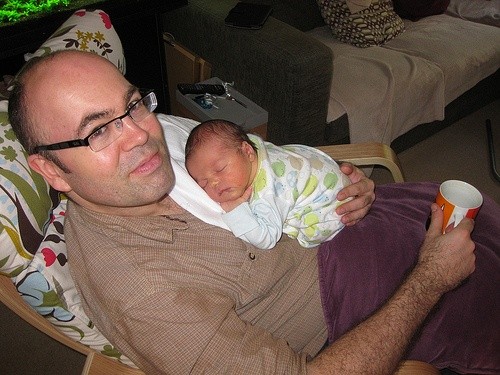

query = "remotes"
[177,83,225,95]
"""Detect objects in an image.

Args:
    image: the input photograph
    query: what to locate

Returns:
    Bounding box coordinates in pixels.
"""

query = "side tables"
[176,78,268,142]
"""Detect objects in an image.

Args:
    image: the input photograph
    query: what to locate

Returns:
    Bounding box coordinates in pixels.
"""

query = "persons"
[186,120,356,250]
[7,49,500,375]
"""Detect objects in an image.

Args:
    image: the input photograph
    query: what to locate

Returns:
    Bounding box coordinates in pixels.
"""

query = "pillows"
[316,0,406,48]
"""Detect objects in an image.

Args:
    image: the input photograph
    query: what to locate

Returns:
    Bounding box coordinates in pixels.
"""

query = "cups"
[436,180,484,234]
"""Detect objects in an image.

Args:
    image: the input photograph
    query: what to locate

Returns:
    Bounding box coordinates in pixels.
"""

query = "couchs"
[176,0,500,148]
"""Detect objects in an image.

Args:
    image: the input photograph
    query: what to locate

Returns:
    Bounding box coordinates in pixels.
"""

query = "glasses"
[26,88,158,153]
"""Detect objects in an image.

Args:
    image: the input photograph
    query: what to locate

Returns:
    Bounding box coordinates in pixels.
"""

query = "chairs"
[1,10,406,375]
